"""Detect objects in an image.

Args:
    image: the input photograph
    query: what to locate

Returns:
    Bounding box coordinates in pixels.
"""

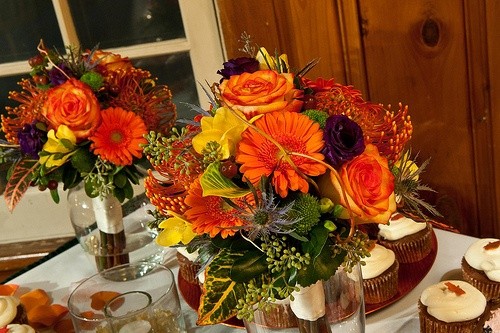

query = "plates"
[177,224,437,329]
[440,268,498,312]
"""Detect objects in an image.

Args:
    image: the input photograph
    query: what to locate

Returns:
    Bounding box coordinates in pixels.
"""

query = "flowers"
[140,32,444,327]
[0,39,177,204]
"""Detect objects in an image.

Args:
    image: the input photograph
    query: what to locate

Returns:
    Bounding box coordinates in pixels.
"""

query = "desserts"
[341,213,500,333]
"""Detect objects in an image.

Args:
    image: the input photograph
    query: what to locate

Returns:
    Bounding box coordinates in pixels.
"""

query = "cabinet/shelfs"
[206,1,499,240]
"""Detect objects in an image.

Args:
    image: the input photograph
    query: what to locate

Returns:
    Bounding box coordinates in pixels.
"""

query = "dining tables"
[0,174,500,333]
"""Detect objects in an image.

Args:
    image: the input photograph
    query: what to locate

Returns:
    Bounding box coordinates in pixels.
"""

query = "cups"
[66,261,187,333]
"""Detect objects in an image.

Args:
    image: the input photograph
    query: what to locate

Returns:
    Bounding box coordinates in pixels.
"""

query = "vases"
[241,251,366,333]
[65,163,168,281]
